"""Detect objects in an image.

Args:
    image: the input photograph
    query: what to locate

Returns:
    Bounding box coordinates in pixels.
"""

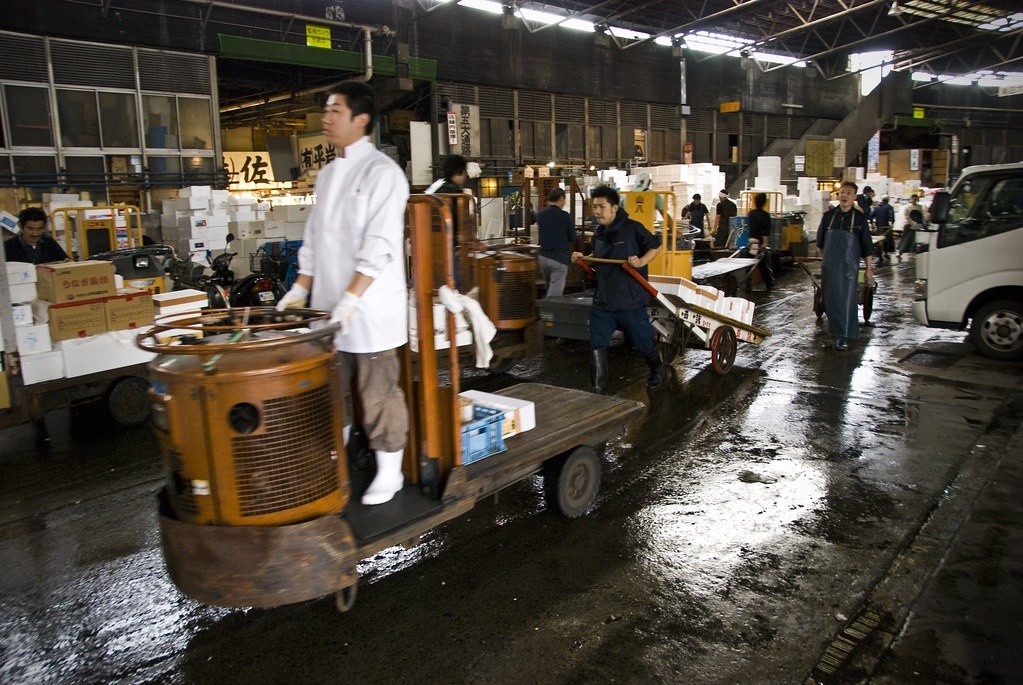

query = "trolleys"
[811,269,878,323]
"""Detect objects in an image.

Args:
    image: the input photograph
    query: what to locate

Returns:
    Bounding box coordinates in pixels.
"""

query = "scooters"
[202,233,279,322]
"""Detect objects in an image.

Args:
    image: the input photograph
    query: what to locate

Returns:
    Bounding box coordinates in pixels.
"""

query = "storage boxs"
[0,156,945,466]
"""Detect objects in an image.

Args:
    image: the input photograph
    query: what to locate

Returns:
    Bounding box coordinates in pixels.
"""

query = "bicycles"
[248,246,313,307]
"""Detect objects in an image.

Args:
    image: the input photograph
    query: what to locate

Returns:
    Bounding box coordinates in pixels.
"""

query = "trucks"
[912,161,1023,361]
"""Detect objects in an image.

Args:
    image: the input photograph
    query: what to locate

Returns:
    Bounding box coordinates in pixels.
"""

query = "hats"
[882,194,890,201]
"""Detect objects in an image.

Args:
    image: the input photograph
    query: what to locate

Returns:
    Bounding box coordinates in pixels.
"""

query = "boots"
[587,344,615,395]
[342,424,351,446]
[361,448,404,505]
[643,344,668,389]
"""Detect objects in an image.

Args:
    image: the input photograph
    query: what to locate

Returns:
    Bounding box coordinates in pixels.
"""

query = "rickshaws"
[574,255,772,376]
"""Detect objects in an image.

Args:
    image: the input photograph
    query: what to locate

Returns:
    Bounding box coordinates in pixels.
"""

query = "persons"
[3,207,71,263]
[746,193,777,292]
[571,186,667,393]
[871,195,895,261]
[856,184,876,219]
[536,188,575,297]
[896,194,924,257]
[816,181,875,350]
[683,194,712,238]
[869,189,874,199]
[618,173,682,238]
[433,155,467,193]
[276,81,410,506]
[711,189,737,247]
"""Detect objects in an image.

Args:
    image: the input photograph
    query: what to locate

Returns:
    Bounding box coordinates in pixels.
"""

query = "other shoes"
[836,336,848,349]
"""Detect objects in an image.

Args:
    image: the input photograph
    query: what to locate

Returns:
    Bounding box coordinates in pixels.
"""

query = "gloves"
[272,284,309,323]
[324,290,362,337]
